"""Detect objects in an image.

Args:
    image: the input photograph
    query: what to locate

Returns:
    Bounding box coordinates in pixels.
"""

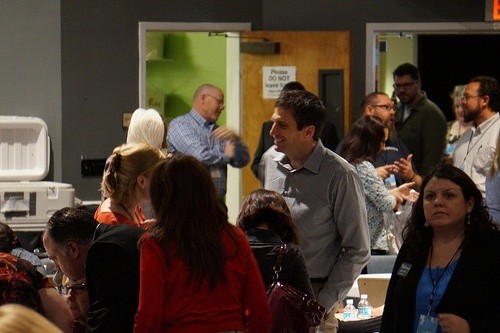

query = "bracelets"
[408,174,416,181]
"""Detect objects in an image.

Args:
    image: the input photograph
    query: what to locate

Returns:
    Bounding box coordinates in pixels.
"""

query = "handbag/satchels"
[267,243,327,333]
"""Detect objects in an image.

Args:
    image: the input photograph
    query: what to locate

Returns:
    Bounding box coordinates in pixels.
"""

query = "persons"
[379,165,500,333]
[336,92,424,193]
[250,82,306,188]
[256,90,371,333]
[128,108,171,218]
[165,83,249,213]
[390,63,448,176]
[134,155,271,333]
[94,143,165,232]
[445,77,500,223]
[236,189,320,333]
[338,114,420,274]
[0,207,146,333]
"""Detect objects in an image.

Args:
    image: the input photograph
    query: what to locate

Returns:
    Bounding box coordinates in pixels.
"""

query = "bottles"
[343,299,357,320]
[358,294,371,319]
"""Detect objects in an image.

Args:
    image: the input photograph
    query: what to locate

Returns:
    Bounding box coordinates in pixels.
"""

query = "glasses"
[370,104,397,112]
[393,81,413,89]
[461,95,481,100]
[202,95,226,105]
